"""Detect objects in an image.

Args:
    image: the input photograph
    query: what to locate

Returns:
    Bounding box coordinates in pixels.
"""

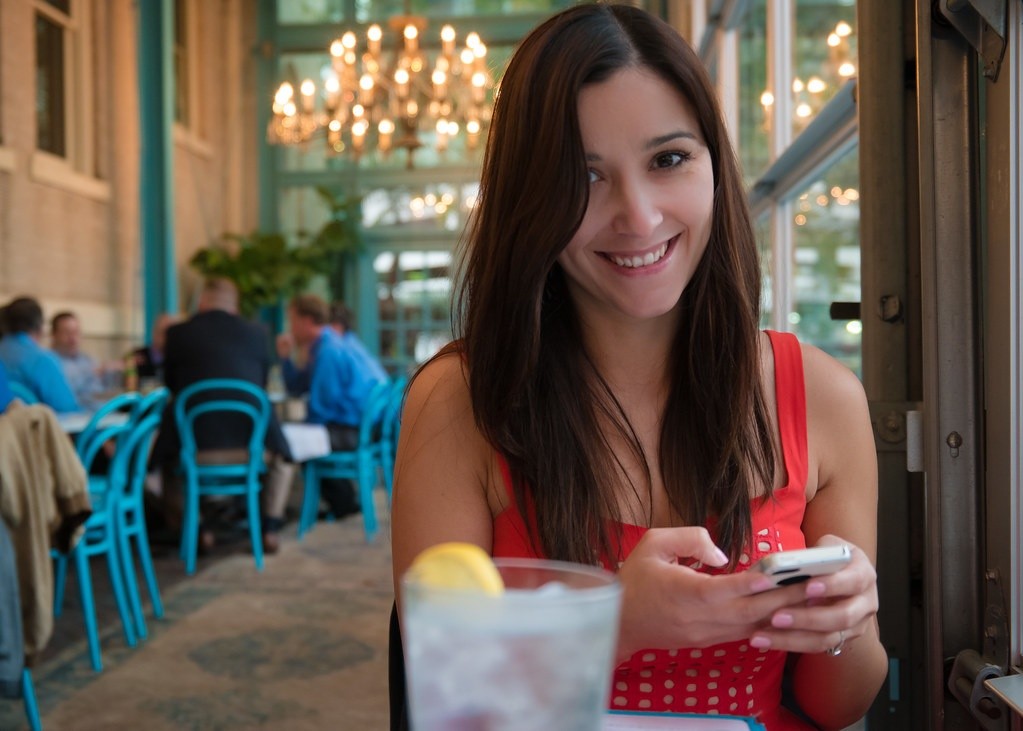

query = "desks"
[59,409,133,432]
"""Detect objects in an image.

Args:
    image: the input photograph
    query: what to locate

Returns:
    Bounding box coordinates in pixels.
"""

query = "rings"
[823,630,845,658]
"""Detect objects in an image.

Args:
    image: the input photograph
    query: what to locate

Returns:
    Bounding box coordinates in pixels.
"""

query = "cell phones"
[749,545,853,587]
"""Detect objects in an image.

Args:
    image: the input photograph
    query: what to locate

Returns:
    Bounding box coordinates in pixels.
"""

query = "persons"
[131,275,273,554]
[49,313,102,400]
[262,296,394,525]
[391,0,887,731]
[1,296,84,416]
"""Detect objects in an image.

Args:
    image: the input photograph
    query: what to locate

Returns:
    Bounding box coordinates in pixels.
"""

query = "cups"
[397,557,622,731]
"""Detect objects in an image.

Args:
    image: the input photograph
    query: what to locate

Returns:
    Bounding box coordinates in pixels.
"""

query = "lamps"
[756,26,861,227]
[273,0,508,170]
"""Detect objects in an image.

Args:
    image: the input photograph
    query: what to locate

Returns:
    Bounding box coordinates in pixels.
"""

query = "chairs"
[0,363,411,731]
